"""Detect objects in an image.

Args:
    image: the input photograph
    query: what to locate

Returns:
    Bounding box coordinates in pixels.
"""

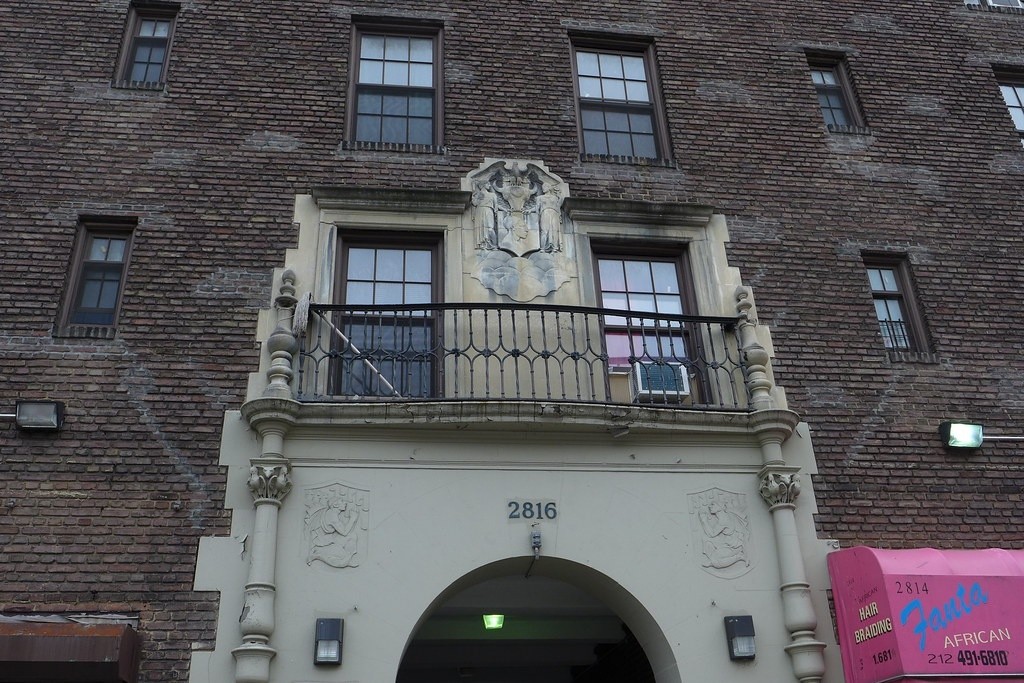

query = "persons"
[523,182,562,254]
[306,495,360,568]
[499,216,529,257]
[699,499,751,568]
[471,178,499,249]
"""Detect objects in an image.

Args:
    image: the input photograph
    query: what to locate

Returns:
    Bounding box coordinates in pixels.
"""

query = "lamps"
[483,613,504,629]
[314,617,344,665]
[15,399,66,432]
[724,615,756,661]
[939,420,984,450]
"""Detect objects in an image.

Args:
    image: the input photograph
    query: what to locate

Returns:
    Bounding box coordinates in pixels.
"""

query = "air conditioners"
[987,0,1023,8]
[627,360,690,403]
